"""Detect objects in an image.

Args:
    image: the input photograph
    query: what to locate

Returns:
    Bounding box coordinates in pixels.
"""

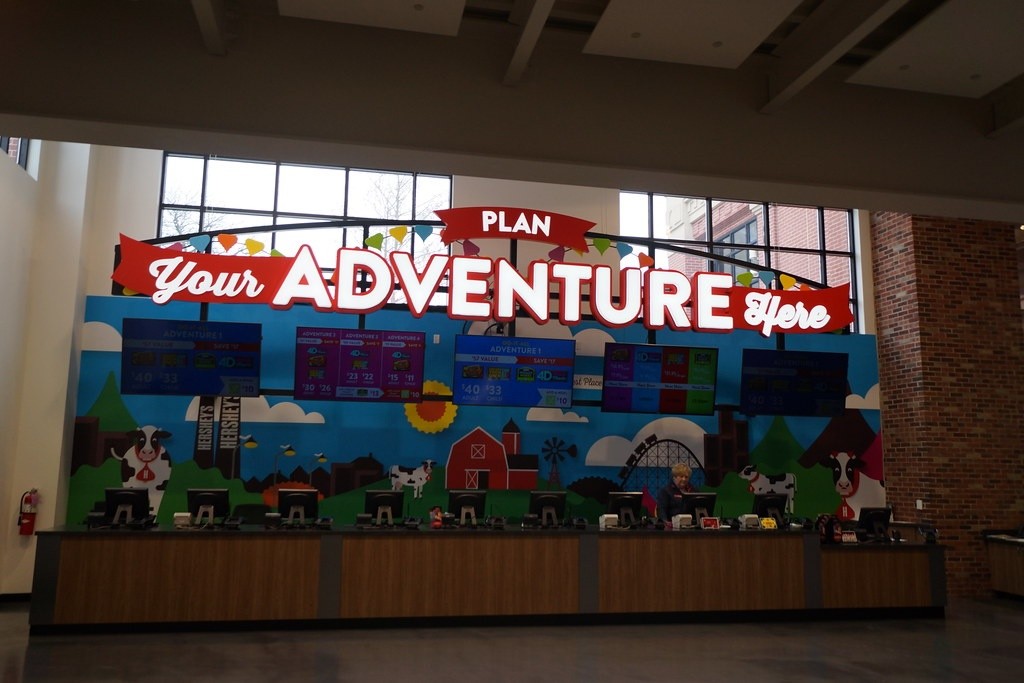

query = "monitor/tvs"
[365,489,404,528]
[859,508,892,543]
[607,491,644,529]
[752,494,787,529]
[278,488,319,529]
[681,492,717,527]
[529,490,567,528]
[188,488,229,529]
[448,489,487,529]
[105,487,149,525]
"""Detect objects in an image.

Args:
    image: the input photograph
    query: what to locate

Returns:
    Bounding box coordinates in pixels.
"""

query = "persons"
[658,462,698,523]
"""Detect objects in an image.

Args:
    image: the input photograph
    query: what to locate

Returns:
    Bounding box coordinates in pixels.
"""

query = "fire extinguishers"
[17,489,39,536]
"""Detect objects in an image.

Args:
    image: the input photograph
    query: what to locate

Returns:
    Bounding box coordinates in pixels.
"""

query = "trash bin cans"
[985,531,1024,601]
